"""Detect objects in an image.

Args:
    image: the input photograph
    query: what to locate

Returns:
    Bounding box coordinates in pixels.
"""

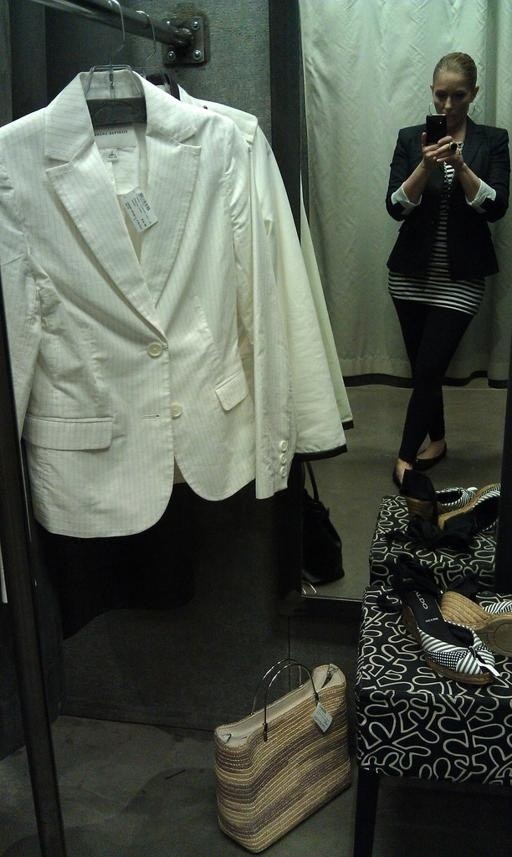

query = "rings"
[449,141,457,153]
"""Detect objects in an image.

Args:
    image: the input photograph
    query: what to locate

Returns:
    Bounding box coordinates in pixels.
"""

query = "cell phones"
[426,114,447,145]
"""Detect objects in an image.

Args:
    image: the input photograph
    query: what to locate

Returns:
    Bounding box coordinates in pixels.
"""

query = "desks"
[356,481,510,855]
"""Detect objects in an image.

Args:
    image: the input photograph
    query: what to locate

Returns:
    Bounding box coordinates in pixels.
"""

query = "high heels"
[405,485,476,529]
[438,589,512,658]
[402,579,498,684]
[437,482,501,546]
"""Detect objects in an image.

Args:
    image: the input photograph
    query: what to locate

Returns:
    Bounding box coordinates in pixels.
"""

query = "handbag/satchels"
[211,657,356,857]
[302,461,347,587]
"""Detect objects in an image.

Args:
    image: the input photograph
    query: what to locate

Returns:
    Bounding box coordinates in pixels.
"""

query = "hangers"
[64,0,160,135]
[131,4,181,109]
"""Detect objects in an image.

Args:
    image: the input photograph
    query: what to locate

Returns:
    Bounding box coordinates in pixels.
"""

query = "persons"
[386,52,510,499]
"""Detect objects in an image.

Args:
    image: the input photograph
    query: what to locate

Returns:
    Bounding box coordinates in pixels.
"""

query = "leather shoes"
[413,439,447,472]
[391,462,423,497]
